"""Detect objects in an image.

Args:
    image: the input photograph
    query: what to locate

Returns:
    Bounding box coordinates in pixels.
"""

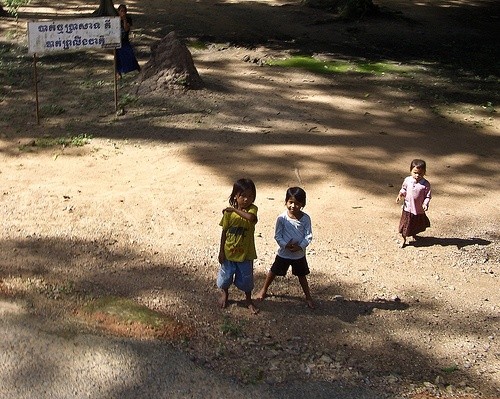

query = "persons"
[217,178,260,314]
[114,3,141,79]
[254,186,317,309]
[395,159,432,248]
